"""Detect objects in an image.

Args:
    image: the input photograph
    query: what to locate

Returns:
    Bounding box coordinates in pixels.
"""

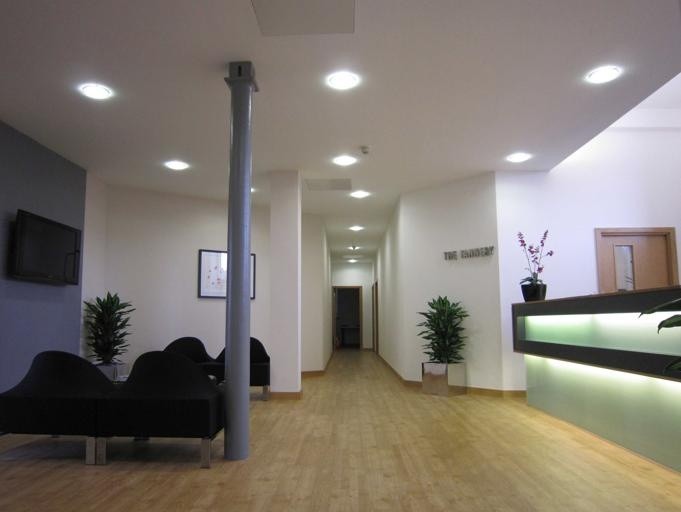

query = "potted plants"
[415,296,468,396]
[81,292,133,382]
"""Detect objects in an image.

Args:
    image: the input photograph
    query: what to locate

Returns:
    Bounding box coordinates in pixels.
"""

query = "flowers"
[511,228,556,284]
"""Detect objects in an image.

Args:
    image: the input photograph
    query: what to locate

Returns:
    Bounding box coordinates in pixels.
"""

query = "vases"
[519,283,548,301]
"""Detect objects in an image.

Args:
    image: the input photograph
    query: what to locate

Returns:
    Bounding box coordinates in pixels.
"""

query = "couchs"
[1,336,270,468]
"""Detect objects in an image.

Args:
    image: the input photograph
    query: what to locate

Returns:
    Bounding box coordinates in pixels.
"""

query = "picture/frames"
[198,249,258,300]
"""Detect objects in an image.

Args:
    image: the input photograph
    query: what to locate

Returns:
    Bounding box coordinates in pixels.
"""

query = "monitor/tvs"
[11,208,81,285]
